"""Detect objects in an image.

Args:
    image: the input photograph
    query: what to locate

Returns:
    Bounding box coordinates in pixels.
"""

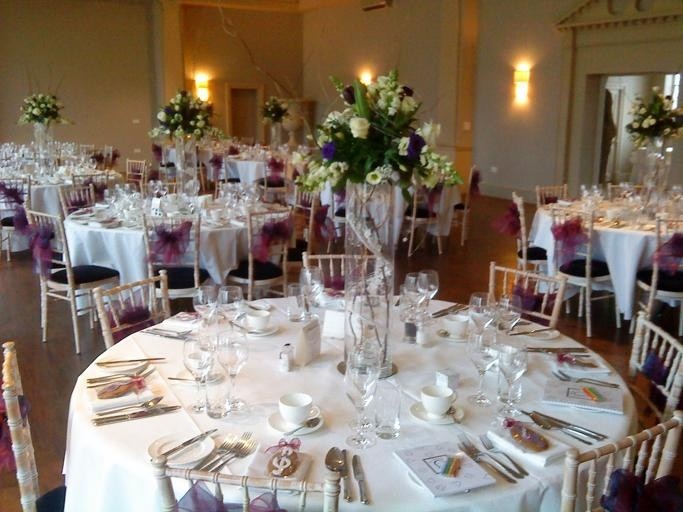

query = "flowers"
[14,92,77,132]
[284,72,464,365]
[624,83,683,182]
[260,97,289,125]
[146,90,225,141]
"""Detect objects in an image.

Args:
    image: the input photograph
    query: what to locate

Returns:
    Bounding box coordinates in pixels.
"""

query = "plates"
[516,325,560,341]
[410,402,466,428]
[267,410,325,436]
[148,434,215,467]
[406,469,424,490]
[99,359,146,372]
[438,329,472,344]
[234,323,282,337]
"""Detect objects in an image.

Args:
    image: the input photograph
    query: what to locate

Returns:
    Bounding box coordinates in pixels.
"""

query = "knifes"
[351,453,371,508]
[95,356,166,365]
[154,428,218,462]
[521,407,607,444]
[525,348,590,354]
[91,406,183,429]
[336,450,353,504]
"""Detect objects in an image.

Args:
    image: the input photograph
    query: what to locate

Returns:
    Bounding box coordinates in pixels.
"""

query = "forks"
[85,360,156,390]
[193,430,260,473]
[550,370,622,390]
[456,431,530,480]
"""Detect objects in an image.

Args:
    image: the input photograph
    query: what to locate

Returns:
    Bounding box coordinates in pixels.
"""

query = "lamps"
[513,67,530,105]
[194,77,209,101]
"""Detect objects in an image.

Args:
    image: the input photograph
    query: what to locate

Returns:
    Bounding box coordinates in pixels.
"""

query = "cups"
[444,314,470,338]
[422,386,458,419]
[288,283,306,324]
[399,286,422,324]
[278,393,321,424]
[373,383,402,440]
[205,370,231,418]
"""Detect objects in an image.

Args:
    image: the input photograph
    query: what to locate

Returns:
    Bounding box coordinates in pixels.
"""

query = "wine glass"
[577,181,683,230]
[193,285,219,335]
[467,327,500,408]
[344,365,373,450]
[216,331,249,411]
[95,178,261,227]
[216,286,245,331]
[405,273,427,304]
[493,345,529,422]
[1,141,97,184]
[181,337,214,415]
[300,266,325,321]
[182,135,309,161]
[347,344,381,430]
[468,292,498,336]
[493,293,523,336]
[417,269,440,309]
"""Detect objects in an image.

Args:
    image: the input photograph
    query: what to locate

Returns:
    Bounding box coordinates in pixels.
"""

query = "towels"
[245,444,313,484]
[487,419,573,468]
[80,371,154,412]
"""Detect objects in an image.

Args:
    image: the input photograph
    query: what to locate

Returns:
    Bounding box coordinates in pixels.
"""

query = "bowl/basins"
[246,310,272,330]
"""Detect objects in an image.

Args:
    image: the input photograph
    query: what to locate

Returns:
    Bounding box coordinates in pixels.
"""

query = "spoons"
[323,446,345,472]
[282,416,320,436]
[447,406,460,424]
[529,411,592,447]
[96,396,165,418]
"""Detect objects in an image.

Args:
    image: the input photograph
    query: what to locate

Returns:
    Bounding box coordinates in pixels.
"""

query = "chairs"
[146,450,342,509]
[18,202,123,356]
[87,269,169,347]
[559,410,679,509]
[2,338,65,512]
[626,307,683,423]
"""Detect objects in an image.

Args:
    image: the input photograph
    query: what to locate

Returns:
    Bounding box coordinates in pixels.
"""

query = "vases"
[268,121,279,152]
[342,168,398,381]
[32,120,49,142]
